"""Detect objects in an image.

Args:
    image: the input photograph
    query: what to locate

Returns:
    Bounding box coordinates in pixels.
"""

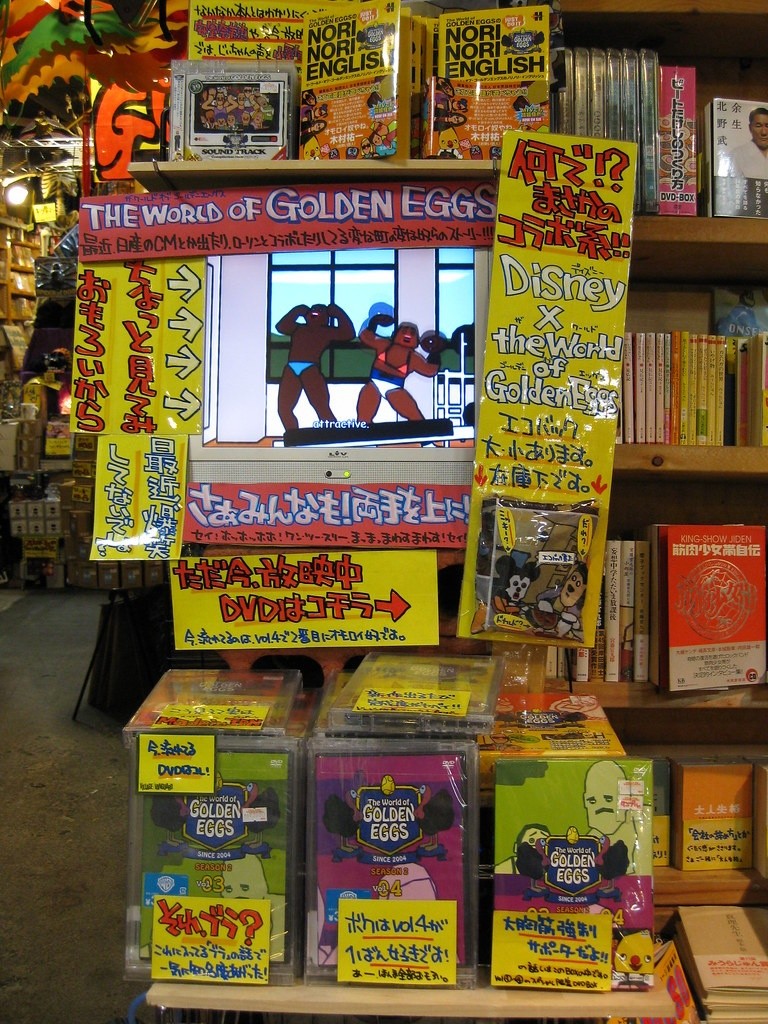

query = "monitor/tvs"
[188,241,495,487]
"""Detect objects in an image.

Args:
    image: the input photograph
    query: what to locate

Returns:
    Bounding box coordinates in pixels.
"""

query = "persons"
[729,108,768,180]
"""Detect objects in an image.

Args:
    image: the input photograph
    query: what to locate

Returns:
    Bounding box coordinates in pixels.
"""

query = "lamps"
[7,171,28,205]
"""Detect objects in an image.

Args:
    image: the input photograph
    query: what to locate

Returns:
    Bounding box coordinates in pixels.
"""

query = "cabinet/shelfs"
[0,216,42,384]
[144,0,768,1017]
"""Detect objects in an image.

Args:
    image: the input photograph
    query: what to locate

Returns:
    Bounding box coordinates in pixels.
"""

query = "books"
[298,0,768,219]
[613,330,768,446]
[542,524,768,692]
[627,755,768,1024]
